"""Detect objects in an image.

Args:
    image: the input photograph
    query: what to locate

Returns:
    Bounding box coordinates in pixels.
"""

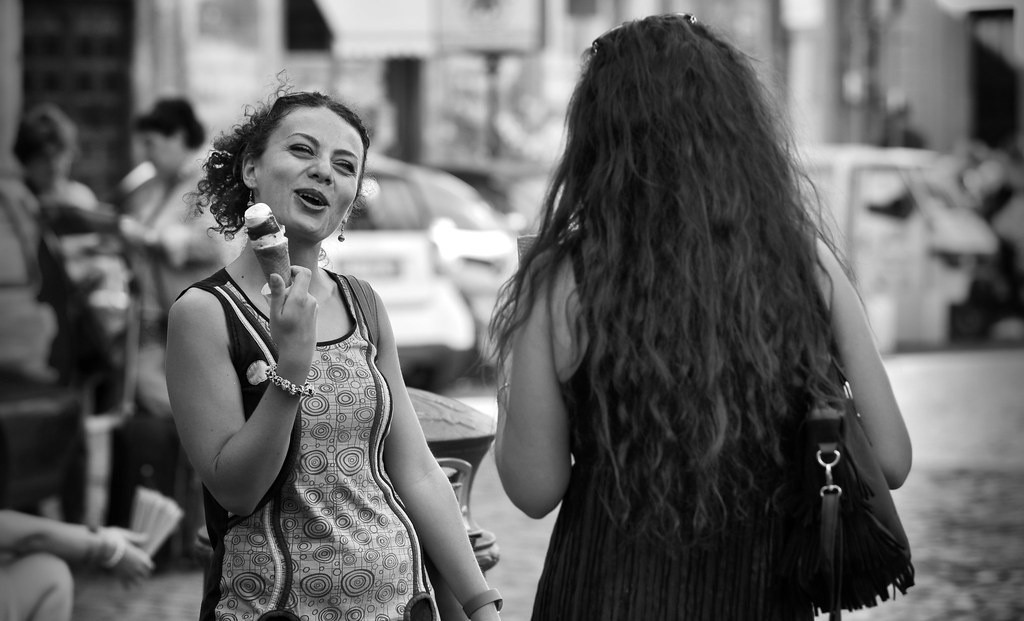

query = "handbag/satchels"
[809,330,915,617]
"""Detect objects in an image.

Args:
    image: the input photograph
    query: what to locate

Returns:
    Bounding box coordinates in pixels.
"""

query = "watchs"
[462,587,503,619]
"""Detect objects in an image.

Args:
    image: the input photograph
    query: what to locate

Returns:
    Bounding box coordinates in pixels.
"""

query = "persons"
[119,96,243,560]
[487,14,913,620]
[1,104,116,385]
[0,507,157,621]
[165,64,506,621]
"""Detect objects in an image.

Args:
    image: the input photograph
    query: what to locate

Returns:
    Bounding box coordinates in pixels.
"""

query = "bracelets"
[85,523,126,569]
[264,363,316,398]
[495,381,511,403]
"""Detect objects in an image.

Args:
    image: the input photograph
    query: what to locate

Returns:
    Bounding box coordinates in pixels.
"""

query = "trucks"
[794,144,1004,350]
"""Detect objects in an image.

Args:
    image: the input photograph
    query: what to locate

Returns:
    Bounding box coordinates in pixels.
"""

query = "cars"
[0,179,86,517]
[114,145,478,395]
[400,160,528,382]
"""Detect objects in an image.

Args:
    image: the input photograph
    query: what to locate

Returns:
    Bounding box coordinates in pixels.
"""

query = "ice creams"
[244,202,292,291]
